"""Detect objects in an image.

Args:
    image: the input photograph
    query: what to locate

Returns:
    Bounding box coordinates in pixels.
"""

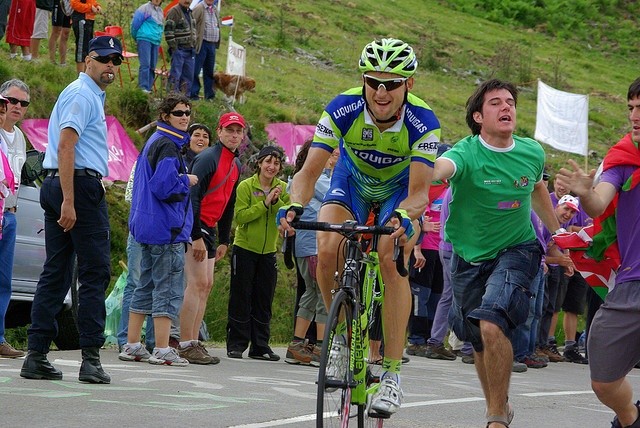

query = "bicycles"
[283,200,408,428]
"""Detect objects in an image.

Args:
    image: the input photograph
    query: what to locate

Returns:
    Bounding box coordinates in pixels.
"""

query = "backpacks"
[22,150,45,186]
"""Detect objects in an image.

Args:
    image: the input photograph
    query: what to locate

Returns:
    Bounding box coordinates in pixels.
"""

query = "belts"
[46,170,101,179]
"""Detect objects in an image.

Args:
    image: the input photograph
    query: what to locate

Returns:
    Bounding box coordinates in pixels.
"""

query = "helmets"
[358,37,418,80]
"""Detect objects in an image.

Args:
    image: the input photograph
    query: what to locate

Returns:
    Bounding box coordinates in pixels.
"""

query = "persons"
[425,185,474,363]
[0,0,36,61]
[535,195,581,361]
[406,143,455,355]
[581,202,605,358]
[130,0,165,93]
[48,0,73,68]
[275,36,442,415]
[182,122,210,166]
[118,90,199,366]
[176,112,246,364]
[284,137,329,367]
[548,175,589,365]
[554,77,640,427]
[366,319,383,366]
[165,0,196,99]
[0,78,30,357]
[30,0,53,58]
[117,159,156,353]
[192,0,221,103]
[402,356,408,363]
[0,92,14,239]
[71,0,103,76]
[512,207,551,374]
[20,35,125,383]
[226,145,281,360]
[431,78,561,428]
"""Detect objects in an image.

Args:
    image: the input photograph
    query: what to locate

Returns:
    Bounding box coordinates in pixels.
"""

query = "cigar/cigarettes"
[419,267,421,272]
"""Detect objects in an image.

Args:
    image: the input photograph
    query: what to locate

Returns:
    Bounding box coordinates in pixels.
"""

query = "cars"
[10,148,83,350]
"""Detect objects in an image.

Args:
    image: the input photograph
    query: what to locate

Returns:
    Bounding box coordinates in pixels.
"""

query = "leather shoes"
[79,347,111,384]
[0,342,24,358]
[20,351,62,380]
[6,341,16,350]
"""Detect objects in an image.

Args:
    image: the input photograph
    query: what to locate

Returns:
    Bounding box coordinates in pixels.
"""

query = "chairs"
[94,30,123,89]
[153,46,170,94]
[103,26,138,80]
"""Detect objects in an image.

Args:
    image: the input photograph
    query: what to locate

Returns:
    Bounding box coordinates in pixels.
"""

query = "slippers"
[368,359,383,365]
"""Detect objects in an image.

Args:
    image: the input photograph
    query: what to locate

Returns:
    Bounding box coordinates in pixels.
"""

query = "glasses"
[363,74,408,91]
[170,109,191,117]
[5,97,29,106]
[91,55,122,66]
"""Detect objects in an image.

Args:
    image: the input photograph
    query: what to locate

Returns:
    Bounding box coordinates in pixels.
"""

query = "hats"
[88,35,124,60]
[257,146,283,159]
[0,93,11,104]
[557,195,580,213]
[218,112,246,128]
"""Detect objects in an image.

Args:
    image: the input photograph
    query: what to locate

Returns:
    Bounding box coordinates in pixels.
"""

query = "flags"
[221,16,234,26]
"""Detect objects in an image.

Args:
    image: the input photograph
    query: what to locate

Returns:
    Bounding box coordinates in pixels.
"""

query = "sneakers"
[536,345,549,362]
[285,339,320,367]
[197,341,220,364]
[309,344,322,355]
[541,345,565,362]
[152,348,190,365]
[118,343,165,363]
[530,354,545,362]
[611,400,640,428]
[512,361,527,372]
[425,342,456,360]
[563,344,589,364]
[227,350,243,358]
[325,334,349,393]
[406,342,426,356]
[520,356,547,368]
[462,355,475,363]
[370,372,404,414]
[402,358,409,363]
[549,340,561,355]
[177,341,216,364]
[248,344,279,361]
